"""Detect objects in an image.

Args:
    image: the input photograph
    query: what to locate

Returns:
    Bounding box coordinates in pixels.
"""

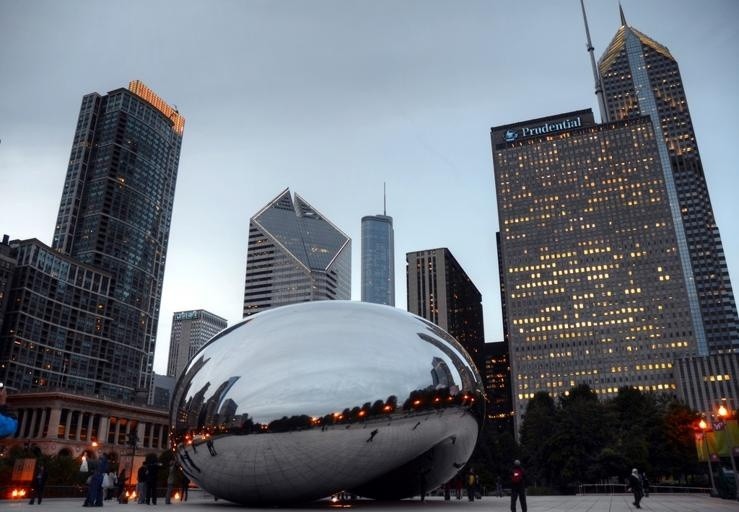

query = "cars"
[658,466,736,492]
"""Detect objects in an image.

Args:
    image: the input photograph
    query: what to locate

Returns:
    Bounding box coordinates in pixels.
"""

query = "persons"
[206,439,214,455]
[184,449,201,472]
[628,465,644,508]
[79,448,190,507]
[191,442,198,453]
[366,427,378,442]
[325,424,328,432]
[208,436,218,455]
[455,475,461,499]
[321,424,324,432]
[642,472,651,497]
[495,475,503,497]
[467,468,476,501]
[29,461,46,505]
[444,482,449,503]
[420,489,426,503]
[508,459,528,512]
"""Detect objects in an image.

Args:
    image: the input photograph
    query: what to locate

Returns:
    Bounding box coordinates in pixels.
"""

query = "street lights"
[717,404,739,498]
[459,394,468,407]
[470,397,475,406]
[697,418,719,497]
